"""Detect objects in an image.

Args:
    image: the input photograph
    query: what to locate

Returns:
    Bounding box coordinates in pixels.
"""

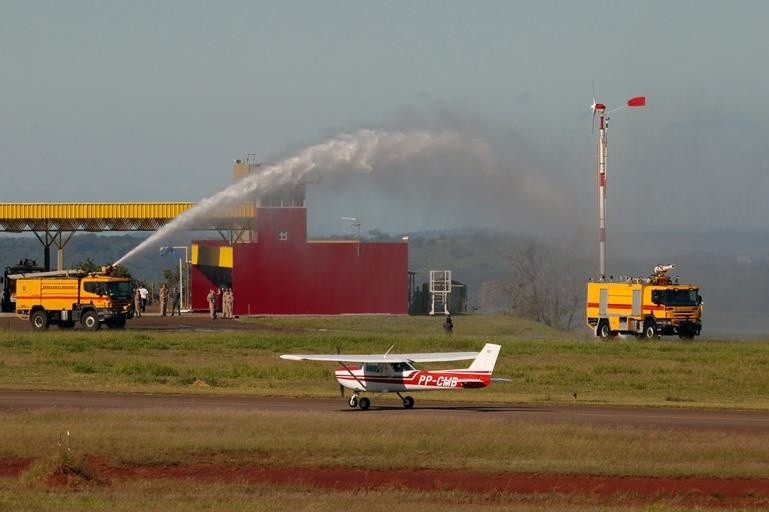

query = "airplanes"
[278,340,502,413]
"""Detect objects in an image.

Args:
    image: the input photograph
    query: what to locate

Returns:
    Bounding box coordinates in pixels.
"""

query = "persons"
[133,285,149,318]
[158,284,168,318]
[443,315,454,333]
[169,287,181,316]
[207,287,235,319]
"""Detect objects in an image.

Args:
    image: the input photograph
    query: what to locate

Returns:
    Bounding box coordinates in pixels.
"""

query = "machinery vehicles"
[2,258,44,313]
[7,267,138,332]
[584,261,705,343]
